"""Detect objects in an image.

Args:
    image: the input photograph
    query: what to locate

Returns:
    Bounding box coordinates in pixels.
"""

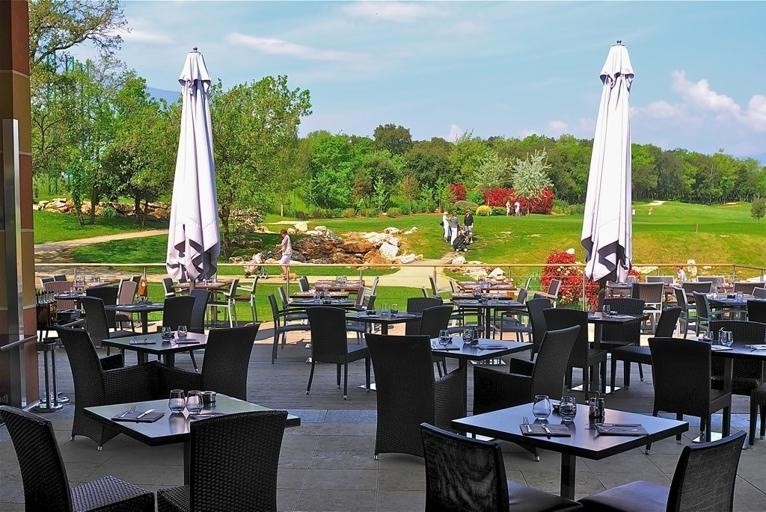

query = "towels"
[666,292,673,302]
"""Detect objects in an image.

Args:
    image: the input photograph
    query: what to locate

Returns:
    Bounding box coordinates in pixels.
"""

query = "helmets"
[699,339,712,342]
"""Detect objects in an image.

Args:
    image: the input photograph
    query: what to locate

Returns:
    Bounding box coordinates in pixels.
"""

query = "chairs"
[0,265,766,510]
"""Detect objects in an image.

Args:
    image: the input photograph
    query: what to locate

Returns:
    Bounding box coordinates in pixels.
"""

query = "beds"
[166,45,220,286]
[581,40,634,293]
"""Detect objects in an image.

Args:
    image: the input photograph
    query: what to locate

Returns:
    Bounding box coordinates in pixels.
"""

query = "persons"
[276,229,293,279]
[506,199,511,215]
[515,200,520,216]
[675,265,687,286]
[443,210,474,252]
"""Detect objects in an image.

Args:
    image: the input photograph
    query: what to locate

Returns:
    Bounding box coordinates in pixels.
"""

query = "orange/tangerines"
[452,224,469,253]
[244,249,276,279]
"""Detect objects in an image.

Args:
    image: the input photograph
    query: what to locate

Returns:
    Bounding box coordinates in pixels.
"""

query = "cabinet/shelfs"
[591,397,605,418]
[202,390,217,401]
[712,292,718,300]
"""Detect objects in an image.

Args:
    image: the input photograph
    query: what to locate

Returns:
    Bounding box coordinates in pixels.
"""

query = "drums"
[136,409,152,418]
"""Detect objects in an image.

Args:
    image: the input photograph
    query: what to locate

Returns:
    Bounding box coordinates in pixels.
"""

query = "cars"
[738,291,743,304]
[464,330,474,344]
[187,391,203,414]
[721,331,736,346]
[533,396,551,418]
[602,305,610,316]
[381,304,389,314]
[161,327,171,341]
[391,304,398,315]
[168,390,185,413]
[561,396,578,422]
[705,331,714,341]
[178,326,187,337]
[439,329,450,345]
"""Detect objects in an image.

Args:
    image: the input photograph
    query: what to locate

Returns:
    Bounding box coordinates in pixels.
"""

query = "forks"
[120,407,133,417]
